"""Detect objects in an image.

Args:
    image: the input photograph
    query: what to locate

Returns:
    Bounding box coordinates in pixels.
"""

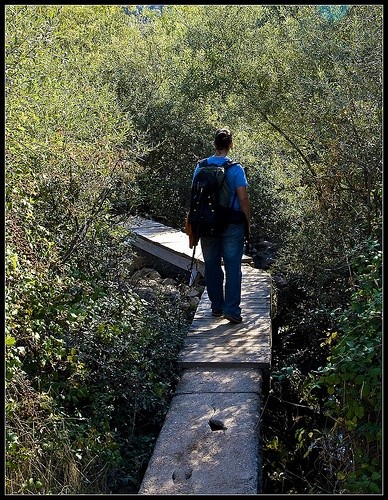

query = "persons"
[185,130,253,322]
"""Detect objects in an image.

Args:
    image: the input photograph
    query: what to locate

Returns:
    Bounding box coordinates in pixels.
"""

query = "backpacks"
[188,158,242,237]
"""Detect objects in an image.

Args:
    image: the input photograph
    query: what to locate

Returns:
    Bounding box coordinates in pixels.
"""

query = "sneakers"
[225,313,243,324]
[211,309,224,316]
